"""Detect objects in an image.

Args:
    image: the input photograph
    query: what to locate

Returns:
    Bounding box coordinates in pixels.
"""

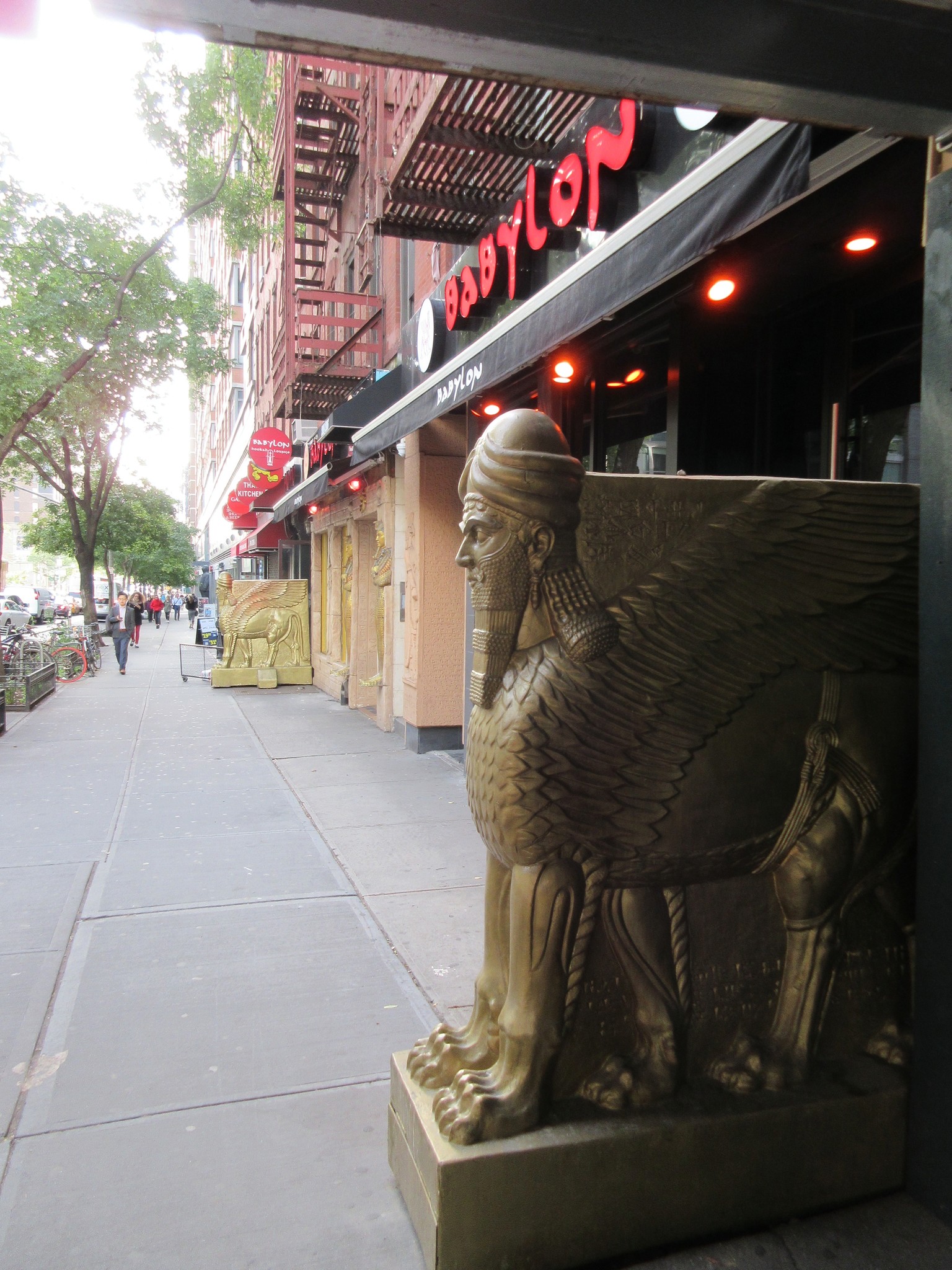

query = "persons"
[108,591,135,674]
[215,571,237,607]
[150,595,164,629]
[199,565,209,604]
[145,593,199,622]
[186,595,197,629]
[127,592,145,648]
[446,395,619,706]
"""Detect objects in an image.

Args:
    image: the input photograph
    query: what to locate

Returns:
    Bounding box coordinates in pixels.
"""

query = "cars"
[0,592,34,636]
[54,596,80,619]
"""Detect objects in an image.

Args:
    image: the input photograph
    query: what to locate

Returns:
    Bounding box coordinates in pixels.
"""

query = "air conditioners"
[290,418,317,446]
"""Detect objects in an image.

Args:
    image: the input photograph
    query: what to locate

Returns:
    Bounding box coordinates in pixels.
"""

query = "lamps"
[351,477,361,490]
[309,504,318,515]
[470,388,504,419]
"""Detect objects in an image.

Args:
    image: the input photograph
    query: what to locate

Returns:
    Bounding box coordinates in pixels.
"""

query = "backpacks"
[105,607,115,637]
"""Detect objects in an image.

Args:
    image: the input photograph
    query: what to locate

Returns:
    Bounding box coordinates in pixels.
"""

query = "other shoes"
[189,623,192,629]
[119,669,126,674]
[156,625,160,629]
[192,627,194,629]
[130,639,135,647]
[135,644,139,648]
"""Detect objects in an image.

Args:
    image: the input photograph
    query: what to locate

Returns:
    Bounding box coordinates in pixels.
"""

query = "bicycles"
[1,620,101,683]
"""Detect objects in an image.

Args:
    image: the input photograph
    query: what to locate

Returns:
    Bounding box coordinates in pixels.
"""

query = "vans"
[3,585,56,626]
[93,580,122,620]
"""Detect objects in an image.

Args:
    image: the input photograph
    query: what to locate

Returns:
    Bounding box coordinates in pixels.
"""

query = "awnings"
[272,455,353,524]
[349,117,814,467]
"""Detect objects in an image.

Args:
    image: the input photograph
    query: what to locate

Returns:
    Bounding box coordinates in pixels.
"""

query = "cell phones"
[120,617,122,619]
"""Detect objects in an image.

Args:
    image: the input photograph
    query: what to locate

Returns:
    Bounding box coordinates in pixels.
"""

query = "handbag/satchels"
[195,609,198,616]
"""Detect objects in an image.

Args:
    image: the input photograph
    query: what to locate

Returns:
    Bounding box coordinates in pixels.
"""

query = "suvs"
[67,591,83,613]
